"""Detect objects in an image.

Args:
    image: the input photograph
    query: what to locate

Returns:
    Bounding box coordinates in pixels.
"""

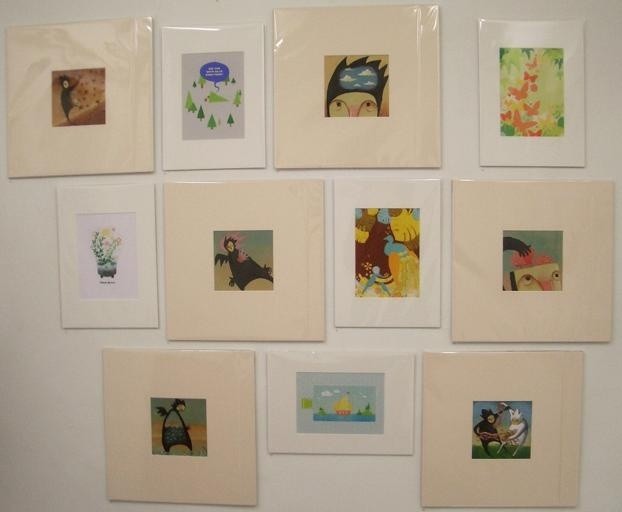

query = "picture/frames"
[6,18,156,178]
[53,183,156,328]
[264,349,413,455]
[272,3,445,169]
[101,351,259,508]
[161,180,327,340]
[477,18,587,166]
[332,177,444,330]
[162,24,264,170]
[419,351,583,510]
[451,178,613,343]
